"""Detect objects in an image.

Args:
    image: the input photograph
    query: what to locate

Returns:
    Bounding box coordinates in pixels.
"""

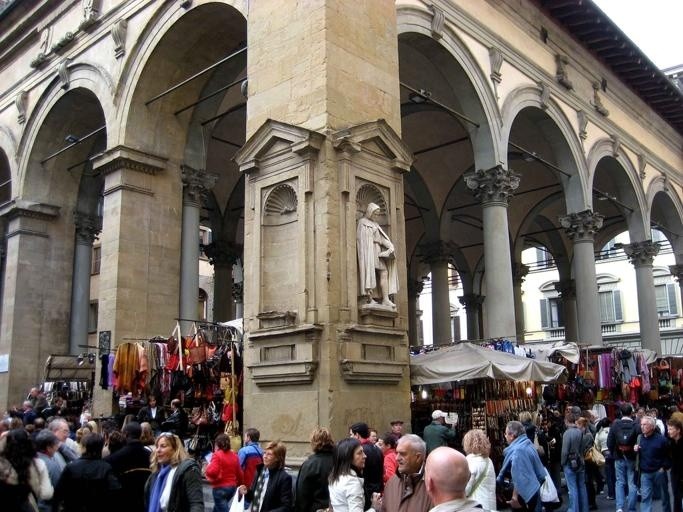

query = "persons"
[357,202,400,309]
[0,380,682,512]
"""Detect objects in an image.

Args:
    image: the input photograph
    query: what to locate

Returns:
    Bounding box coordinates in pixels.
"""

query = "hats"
[432,410,448,420]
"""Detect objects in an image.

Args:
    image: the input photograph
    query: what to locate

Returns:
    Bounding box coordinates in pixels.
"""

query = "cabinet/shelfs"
[470,378,537,465]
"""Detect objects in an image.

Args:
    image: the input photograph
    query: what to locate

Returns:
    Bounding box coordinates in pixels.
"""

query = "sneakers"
[600,490,614,500]
[616,509,624,512]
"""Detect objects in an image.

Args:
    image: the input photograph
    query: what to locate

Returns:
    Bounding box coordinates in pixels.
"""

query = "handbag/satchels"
[168,336,242,452]
[584,446,606,466]
[568,452,582,470]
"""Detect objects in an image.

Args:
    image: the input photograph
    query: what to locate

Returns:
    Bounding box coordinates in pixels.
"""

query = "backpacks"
[613,420,637,460]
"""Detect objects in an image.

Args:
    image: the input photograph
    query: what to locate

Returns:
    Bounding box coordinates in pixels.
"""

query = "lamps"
[64,134,78,143]
[597,192,616,201]
[408,89,432,102]
[76,352,96,366]
[522,151,539,163]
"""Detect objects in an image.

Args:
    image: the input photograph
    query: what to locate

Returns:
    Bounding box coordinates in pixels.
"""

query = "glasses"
[159,432,174,442]
